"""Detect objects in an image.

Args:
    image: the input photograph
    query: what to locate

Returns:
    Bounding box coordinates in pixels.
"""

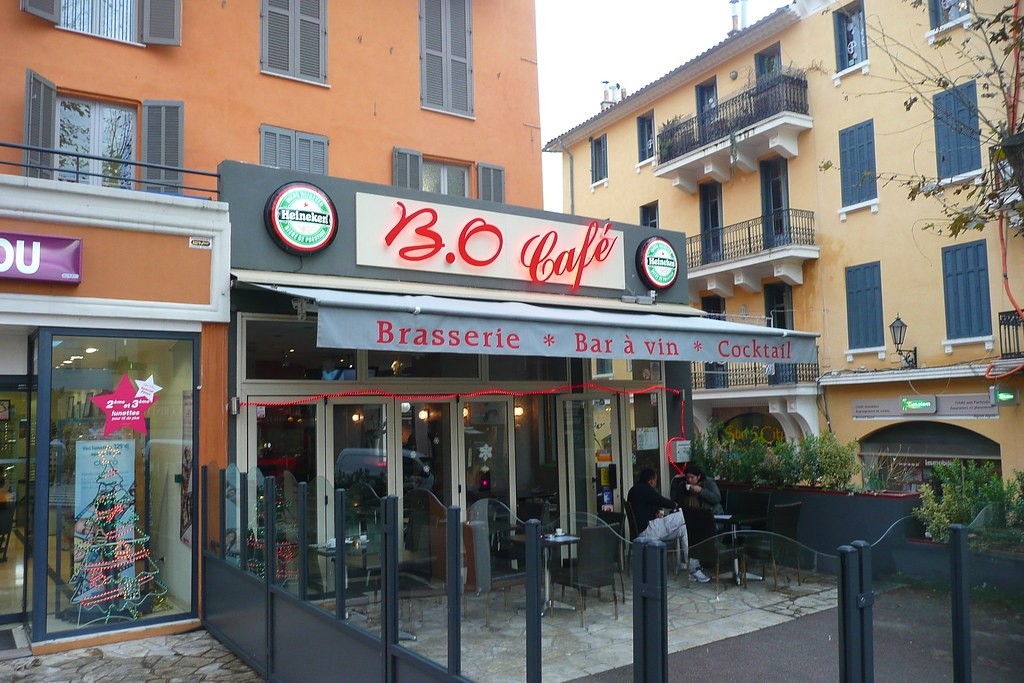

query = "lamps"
[889,313,919,368]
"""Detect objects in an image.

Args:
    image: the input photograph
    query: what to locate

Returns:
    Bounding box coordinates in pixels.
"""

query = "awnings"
[230,271,820,368]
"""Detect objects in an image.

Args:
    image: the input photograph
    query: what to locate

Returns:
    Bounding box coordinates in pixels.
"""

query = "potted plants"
[657,60,826,181]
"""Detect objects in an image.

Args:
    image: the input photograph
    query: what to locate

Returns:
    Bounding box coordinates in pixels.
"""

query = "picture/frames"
[76,402,81,419]
[83,391,93,417]
[67,395,74,417]
[0,399,11,421]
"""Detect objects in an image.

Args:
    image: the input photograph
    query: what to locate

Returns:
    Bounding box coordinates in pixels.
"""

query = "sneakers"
[681,563,687,569]
[689,570,710,582]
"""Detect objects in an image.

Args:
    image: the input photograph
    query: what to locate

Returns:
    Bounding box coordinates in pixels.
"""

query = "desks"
[499,532,583,616]
[714,512,771,584]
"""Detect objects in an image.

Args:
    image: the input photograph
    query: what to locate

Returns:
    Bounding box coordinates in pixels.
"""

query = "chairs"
[685,507,746,599]
[744,491,771,529]
[579,510,627,605]
[738,501,801,591]
[550,523,619,625]
[701,480,729,513]
[484,520,525,625]
[622,498,675,577]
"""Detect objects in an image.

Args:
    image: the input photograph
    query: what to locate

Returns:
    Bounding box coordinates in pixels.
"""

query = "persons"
[627,468,710,583]
[674,467,725,530]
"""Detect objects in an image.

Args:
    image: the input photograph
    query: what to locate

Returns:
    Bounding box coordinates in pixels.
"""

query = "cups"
[685,484,691,491]
[555,528,562,535]
[326,538,335,549]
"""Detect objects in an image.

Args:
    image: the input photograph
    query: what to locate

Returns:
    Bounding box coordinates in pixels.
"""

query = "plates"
[555,533,565,536]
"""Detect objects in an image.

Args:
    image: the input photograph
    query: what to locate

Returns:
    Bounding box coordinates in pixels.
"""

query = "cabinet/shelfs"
[55,417,151,618]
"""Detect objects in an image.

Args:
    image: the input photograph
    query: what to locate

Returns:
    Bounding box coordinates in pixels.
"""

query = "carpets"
[0,624,34,660]
